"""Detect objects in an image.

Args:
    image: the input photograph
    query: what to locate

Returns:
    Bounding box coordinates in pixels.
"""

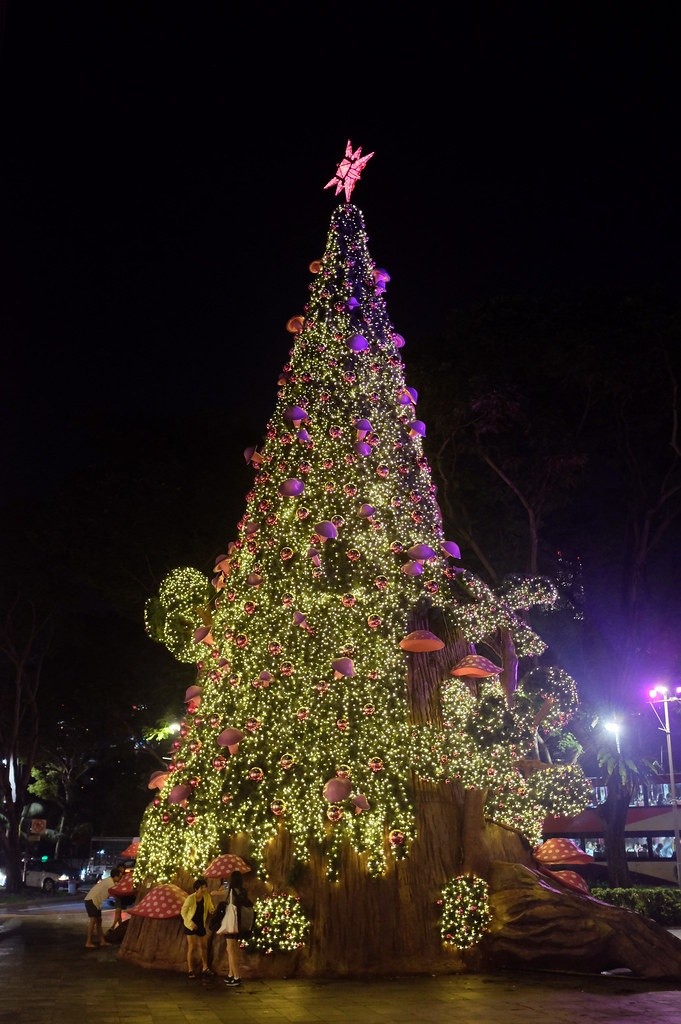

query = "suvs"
[20,861,85,892]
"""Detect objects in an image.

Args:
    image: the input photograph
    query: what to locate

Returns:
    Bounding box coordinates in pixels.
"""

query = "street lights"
[646,685,681,887]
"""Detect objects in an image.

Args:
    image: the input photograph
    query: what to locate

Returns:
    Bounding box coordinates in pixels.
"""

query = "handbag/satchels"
[208,902,226,931]
[216,888,239,934]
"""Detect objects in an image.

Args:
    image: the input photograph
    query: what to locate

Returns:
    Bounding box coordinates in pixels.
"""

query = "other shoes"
[226,978,241,987]
[187,971,196,979]
[201,969,214,977]
[100,942,114,947]
[85,943,97,948]
[224,976,234,983]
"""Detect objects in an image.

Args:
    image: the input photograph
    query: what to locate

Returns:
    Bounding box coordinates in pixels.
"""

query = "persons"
[107,863,126,934]
[657,794,663,805]
[84,869,121,949]
[636,840,663,857]
[210,872,248,986]
[180,880,216,978]
[586,841,602,856]
[96,861,106,880]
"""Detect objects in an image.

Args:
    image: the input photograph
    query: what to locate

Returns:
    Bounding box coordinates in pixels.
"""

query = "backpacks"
[235,893,254,932]
